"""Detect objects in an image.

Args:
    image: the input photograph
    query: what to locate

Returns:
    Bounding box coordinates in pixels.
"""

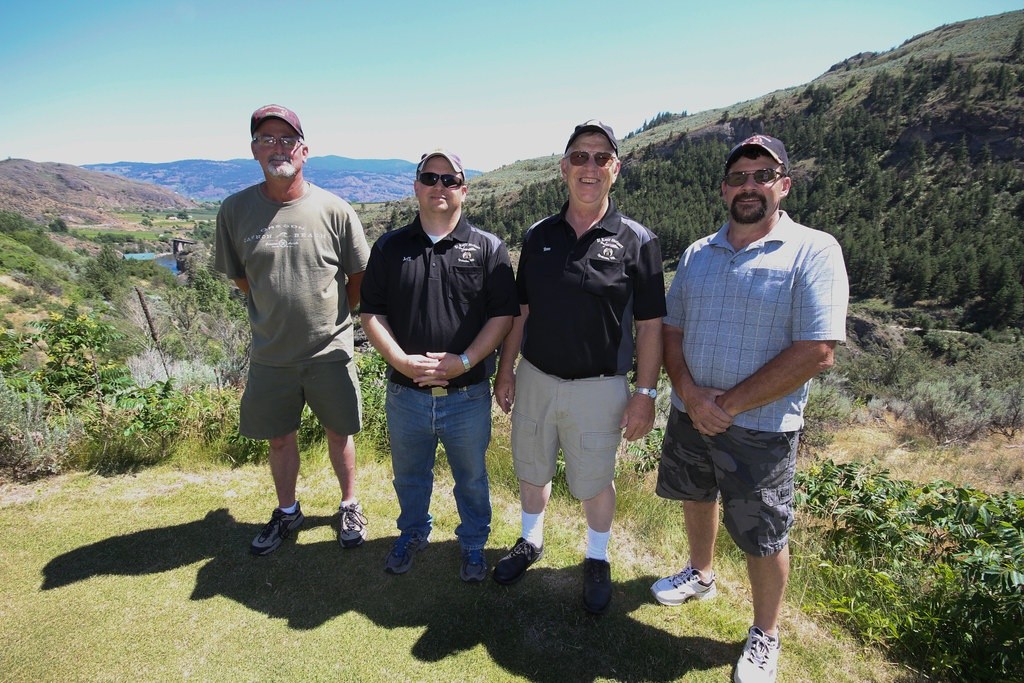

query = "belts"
[390,374,471,397]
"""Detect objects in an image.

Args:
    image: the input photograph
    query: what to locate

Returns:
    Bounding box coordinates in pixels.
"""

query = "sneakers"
[385,532,428,574]
[650,559,717,605]
[250,501,303,555]
[734,626,782,683]
[493,537,543,586]
[457,536,488,582]
[339,503,371,548]
[581,557,612,614]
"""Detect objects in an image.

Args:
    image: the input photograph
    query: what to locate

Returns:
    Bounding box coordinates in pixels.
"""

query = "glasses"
[724,168,783,188]
[419,172,464,190]
[253,135,303,149]
[565,150,616,167]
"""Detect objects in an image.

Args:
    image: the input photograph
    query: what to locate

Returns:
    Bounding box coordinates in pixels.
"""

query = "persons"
[651,134,849,683]
[360,149,522,583]
[212,103,371,555]
[489,120,667,615]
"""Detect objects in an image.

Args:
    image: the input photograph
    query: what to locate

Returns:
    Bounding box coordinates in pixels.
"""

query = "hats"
[725,134,789,177]
[565,119,619,157]
[251,104,305,140]
[416,146,466,181]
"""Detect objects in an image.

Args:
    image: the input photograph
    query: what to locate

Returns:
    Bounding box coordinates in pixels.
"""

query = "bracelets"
[458,353,470,374]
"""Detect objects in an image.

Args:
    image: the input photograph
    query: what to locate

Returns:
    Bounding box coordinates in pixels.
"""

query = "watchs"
[634,387,658,399]
